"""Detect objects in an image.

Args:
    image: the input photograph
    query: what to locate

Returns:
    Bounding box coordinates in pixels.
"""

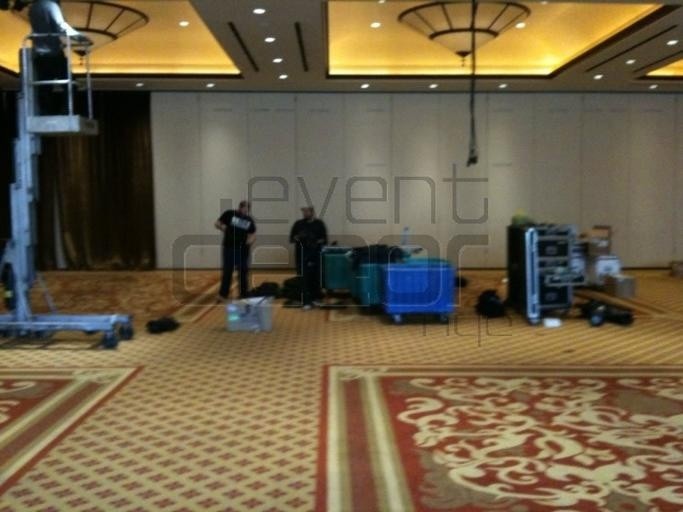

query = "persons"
[27,1,94,119]
[286,203,331,306]
[211,199,259,302]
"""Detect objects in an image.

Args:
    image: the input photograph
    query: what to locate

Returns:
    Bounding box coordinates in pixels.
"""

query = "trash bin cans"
[319,244,457,324]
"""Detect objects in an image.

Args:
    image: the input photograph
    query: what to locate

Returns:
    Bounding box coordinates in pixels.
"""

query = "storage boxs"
[582,233,637,301]
[322,247,455,324]
[225,298,273,334]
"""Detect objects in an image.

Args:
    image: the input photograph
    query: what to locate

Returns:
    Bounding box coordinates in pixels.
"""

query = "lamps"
[397,1,531,65]
[10,0,149,66]
